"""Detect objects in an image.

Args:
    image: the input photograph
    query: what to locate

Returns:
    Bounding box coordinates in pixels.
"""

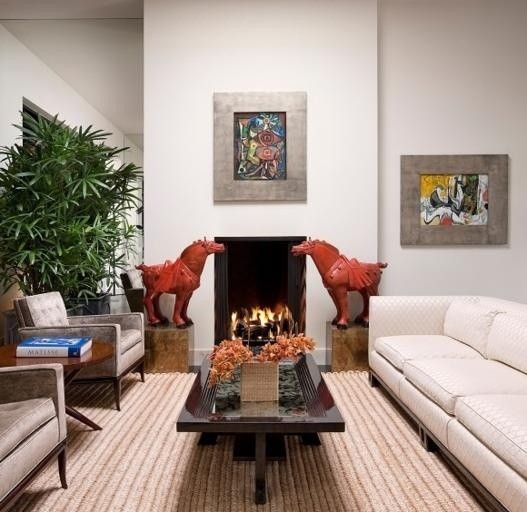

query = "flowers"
[207,333,316,390]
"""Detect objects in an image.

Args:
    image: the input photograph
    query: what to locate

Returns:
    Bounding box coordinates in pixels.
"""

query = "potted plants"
[1,110,149,317]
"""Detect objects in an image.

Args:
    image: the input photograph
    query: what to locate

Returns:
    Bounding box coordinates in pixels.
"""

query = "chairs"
[0,362,70,511]
[13,290,145,412]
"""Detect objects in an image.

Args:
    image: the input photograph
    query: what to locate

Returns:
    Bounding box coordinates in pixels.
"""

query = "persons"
[428,184,451,208]
[450,181,464,215]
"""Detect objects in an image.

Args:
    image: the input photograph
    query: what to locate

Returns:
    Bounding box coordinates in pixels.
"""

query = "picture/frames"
[398,153,508,246]
[214,91,308,203]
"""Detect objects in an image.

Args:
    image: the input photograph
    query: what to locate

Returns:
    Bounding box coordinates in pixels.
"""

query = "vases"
[240,361,279,403]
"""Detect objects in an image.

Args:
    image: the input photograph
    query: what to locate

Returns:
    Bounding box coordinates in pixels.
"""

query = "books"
[14,337,94,359]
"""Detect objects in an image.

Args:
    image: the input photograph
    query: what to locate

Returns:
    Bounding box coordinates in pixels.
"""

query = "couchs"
[367,294,527,512]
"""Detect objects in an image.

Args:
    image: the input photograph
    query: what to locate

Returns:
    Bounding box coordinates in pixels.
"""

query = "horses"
[134,236,226,330]
[290,235,388,330]
